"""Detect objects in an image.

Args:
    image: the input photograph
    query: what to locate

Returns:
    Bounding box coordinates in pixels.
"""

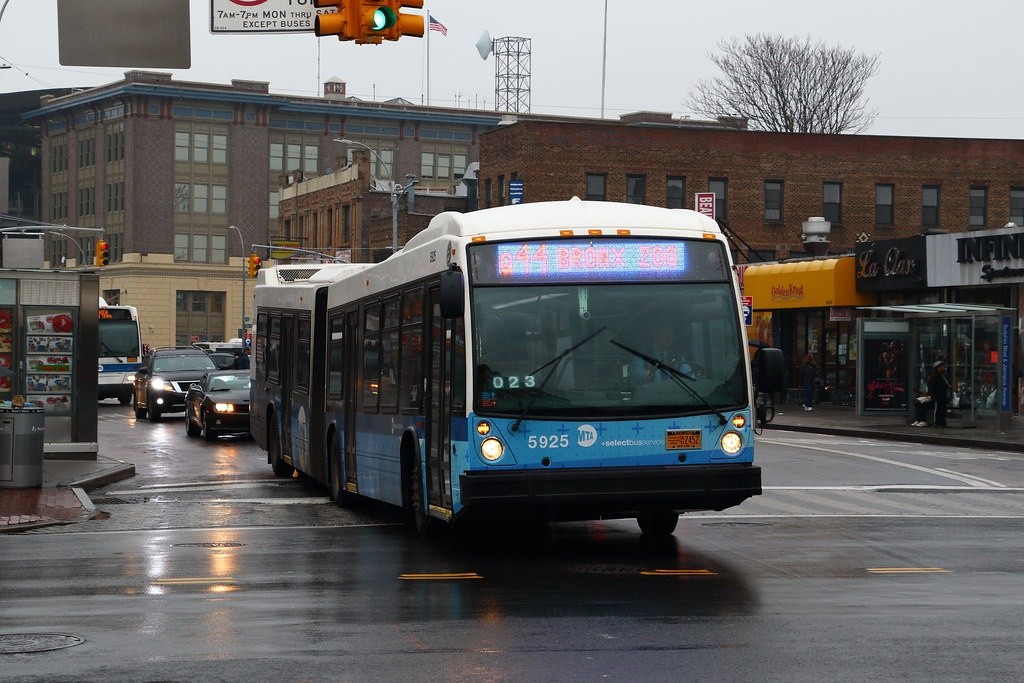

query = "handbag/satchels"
[959,389,971,408]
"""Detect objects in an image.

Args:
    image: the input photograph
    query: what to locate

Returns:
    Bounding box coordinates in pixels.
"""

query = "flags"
[430,15,448,37]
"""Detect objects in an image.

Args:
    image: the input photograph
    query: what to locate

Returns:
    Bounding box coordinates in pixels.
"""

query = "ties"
[660,352,666,380]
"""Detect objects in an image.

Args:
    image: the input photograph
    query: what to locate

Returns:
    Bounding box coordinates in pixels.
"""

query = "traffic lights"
[96,242,109,267]
[248,256,261,278]
[314,0,425,38]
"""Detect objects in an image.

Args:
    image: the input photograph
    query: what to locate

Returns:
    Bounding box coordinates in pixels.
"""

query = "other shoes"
[916,421,928,427]
[911,421,920,426]
[807,406,812,412]
[803,404,807,410]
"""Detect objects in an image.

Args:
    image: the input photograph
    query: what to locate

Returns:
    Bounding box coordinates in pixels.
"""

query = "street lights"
[229,225,245,356]
[48,230,86,270]
[332,137,420,255]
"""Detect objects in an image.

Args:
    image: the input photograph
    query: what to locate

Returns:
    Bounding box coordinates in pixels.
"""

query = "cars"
[184,370,251,439]
[206,350,238,370]
[133,345,220,420]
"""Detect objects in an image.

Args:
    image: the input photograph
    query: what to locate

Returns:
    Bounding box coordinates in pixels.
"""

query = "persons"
[234,346,251,370]
[911,360,953,428]
[631,322,701,388]
[800,354,819,411]
[881,341,904,380]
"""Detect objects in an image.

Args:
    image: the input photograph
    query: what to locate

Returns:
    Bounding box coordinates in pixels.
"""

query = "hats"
[933,361,942,367]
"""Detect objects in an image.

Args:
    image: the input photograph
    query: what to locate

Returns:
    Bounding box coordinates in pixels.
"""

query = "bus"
[193,338,243,356]
[98,297,144,405]
[247,195,786,540]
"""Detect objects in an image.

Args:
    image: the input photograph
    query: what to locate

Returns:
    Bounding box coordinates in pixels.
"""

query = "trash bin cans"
[0,401,46,490]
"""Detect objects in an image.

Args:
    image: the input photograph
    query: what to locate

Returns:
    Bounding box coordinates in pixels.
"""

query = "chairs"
[212,379,225,389]
